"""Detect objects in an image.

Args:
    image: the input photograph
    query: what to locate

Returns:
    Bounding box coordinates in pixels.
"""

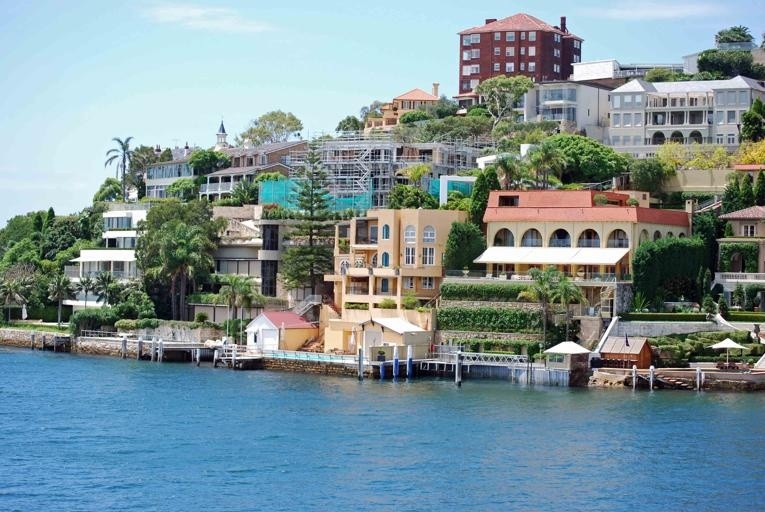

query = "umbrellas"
[707,338,750,350]
[712,338,746,369]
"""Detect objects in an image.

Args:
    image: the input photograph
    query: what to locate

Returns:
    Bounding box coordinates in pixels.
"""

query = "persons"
[223,339,229,352]
[440,341,446,362]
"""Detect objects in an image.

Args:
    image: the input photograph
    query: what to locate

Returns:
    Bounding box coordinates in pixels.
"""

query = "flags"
[625,332,630,347]
